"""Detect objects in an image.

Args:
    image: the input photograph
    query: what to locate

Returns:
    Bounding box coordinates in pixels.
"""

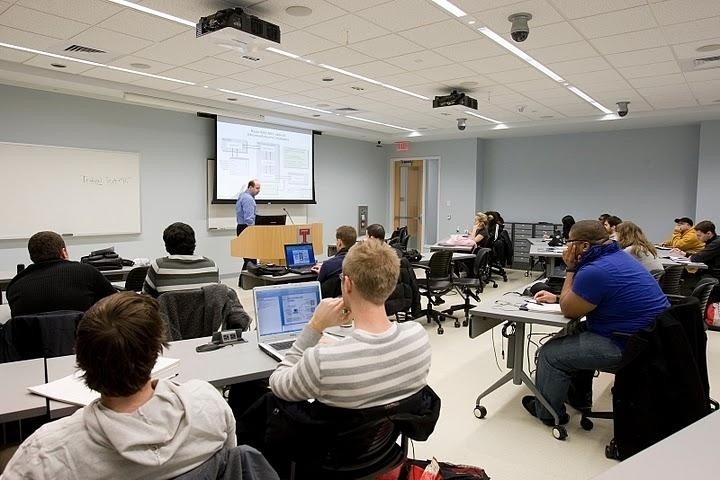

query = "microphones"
[283,208,293,224]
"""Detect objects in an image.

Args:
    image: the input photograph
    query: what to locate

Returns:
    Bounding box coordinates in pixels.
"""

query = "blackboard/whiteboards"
[0,142,142,241]
[206,158,308,231]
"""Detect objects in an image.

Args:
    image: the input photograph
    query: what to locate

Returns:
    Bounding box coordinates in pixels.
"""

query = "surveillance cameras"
[377,140,381,144]
[510,20,529,42]
[617,103,628,116]
[458,120,465,130]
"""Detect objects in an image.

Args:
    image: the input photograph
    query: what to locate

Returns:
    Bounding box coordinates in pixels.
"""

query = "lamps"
[507,12,532,42]
[616,102,630,116]
[456,118,467,130]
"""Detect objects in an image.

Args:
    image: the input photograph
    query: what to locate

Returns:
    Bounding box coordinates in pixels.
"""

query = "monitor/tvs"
[255,215,286,225]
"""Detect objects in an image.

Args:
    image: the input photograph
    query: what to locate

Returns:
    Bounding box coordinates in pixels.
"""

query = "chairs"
[580,265,720,431]
[17,263,24,274]
[322,228,511,334]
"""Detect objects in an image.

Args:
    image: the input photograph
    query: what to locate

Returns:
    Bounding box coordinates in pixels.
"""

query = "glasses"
[565,239,591,246]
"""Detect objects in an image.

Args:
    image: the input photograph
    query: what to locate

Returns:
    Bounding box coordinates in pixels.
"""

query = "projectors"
[433,95,477,112]
[196,8,280,50]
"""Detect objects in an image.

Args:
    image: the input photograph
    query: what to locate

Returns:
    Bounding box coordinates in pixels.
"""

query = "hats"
[674,217,693,226]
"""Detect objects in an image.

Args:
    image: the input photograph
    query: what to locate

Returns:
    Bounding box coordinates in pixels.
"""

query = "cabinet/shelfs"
[502,221,563,271]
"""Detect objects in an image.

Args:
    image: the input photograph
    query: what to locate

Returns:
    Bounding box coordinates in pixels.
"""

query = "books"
[28,355,181,407]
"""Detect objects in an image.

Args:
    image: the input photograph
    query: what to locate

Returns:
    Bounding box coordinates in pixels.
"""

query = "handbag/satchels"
[377,458,490,479]
[80,247,122,272]
[246,261,283,276]
[705,302,720,327]
[447,234,475,247]
[405,248,422,262]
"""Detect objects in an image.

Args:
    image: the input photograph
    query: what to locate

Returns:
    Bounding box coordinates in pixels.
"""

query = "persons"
[523,220,671,426]
[0,291,240,479]
[236,179,260,287]
[5,231,119,319]
[454,213,720,304]
[311,226,357,283]
[268,238,429,480]
[366,224,403,258]
[141,222,220,299]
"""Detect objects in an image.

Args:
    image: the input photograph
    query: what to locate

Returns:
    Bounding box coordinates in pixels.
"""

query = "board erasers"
[62,234,73,236]
[210,228,217,229]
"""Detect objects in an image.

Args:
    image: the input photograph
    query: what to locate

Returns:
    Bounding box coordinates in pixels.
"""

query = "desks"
[239,259,324,290]
[100,263,139,275]
[592,408,720,480]
[648,240,708,269]
[468,237,592,439]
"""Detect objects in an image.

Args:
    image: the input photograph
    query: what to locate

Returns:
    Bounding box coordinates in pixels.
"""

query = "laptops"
[284,243,321,274]
[252,281,342,362]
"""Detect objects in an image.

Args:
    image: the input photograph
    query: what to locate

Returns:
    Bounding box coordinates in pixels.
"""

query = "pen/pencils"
[168,374,178,380]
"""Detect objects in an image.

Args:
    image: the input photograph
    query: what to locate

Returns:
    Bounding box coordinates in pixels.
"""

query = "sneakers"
[522,396,570,425]
